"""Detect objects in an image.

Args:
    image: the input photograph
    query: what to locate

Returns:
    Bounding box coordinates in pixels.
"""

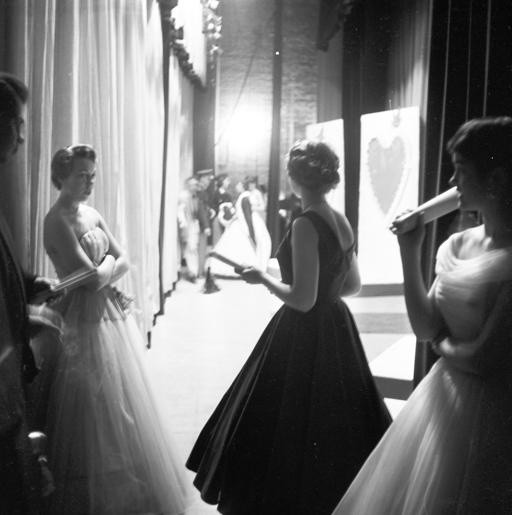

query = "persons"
[177,166,272,285]
[1,72,60,513]
[326,113,511,515]
[26,144,192,515]
[183,136,392,515]
